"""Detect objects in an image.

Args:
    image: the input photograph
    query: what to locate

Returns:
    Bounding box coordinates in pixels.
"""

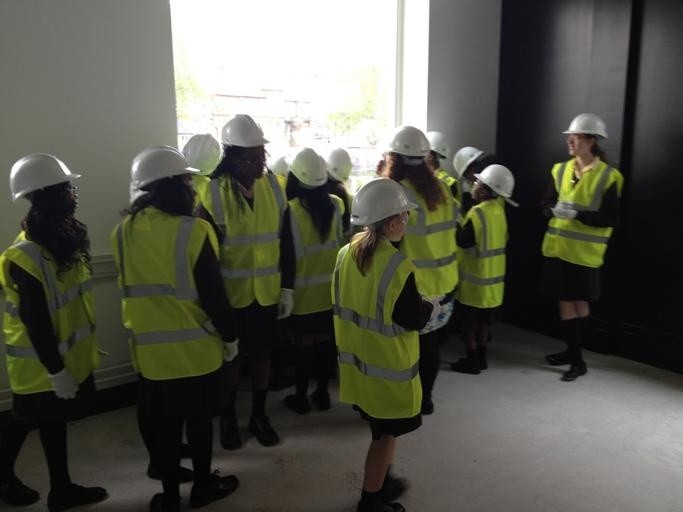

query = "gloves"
[424,294,444,322]
[224,339,240,363]
[48,366,80,401]
[276,288,296,321]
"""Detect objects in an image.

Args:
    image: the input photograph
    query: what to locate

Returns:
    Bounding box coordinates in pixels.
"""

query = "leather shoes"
[147,459,195,486]
[561,362,588,381]
[47,486,108,510]
[451,360,481,375]
[188,468,239,509]
[284,393,311,415]
[459,355,487,371]
[356,500,403,512]
[421,395,436,417]
[248,414,278,448]
[386,478,405,497]
[1,474,41,508]
[151,491,180,511]
[310,390,332,411]
[220,414,243,451]
[546,352,572,365]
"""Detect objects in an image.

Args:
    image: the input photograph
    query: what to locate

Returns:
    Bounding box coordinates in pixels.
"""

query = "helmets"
[560,113,609,139]
[423,131,453,161]
[130,145,202,192]
[453,146,484,181]
[288,148,329,188]
[473,164,515,199]
[389,126,431,158]
[10,153,81,204]
[182,134,221,176]
[271,157,289,177]
[221,115,270,148]
[350,180,419,227]
[326,148,353,183]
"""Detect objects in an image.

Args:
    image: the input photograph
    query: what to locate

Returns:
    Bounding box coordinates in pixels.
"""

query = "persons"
[182,133,220,206]
[332,178,445,512]
[541,112,623,382]
[195,114,295,449]
[451,164,519,375]
[425,132,462,205]
[452,146,503,215]
[112,144,238,512]
[266,155,293,192]
[376,125,460,414]
[324,147,365,240]
[0,152,109,512]
[282,149,344,414]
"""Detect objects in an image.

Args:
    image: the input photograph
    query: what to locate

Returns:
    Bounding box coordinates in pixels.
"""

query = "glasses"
[401,214,409,225]
[231,153,267,166]
[563,135,585,143]
[54,185,80,199]
[472,180,487,194]
